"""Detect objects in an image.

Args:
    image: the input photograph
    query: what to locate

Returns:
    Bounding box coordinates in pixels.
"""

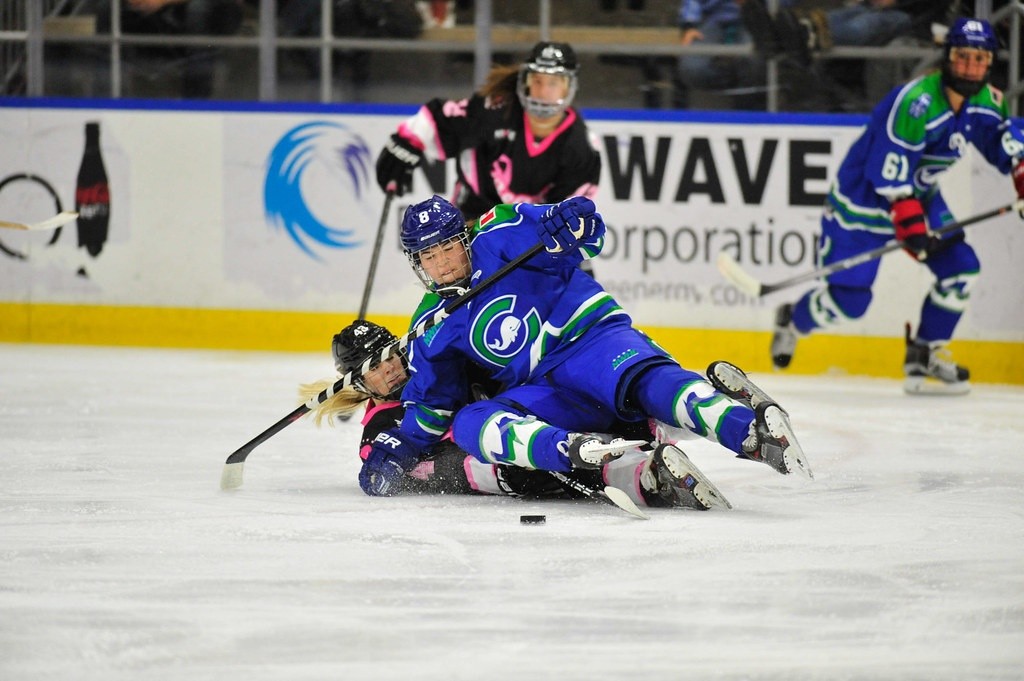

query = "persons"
[377,44,601,216]
[673,0,1023,114]
[355,193,822,511]
[771,20,1024,392]
[334,317,731,509]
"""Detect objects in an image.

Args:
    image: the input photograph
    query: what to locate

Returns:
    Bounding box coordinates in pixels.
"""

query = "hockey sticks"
[215,243,549,492]
[337,179,397,421]
[716,198,1024,299]
[546,468,651,520]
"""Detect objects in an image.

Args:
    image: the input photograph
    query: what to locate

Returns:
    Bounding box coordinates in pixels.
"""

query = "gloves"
[891,197,966,263]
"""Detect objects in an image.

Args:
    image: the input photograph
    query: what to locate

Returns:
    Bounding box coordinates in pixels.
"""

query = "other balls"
[520,515,546,526]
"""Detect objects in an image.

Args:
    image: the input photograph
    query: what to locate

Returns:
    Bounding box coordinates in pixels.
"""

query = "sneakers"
[734,402,815,487]
[576,426,650,468]
[768,300,801,369]
[902,322,972,397]
[707,359,790,428]
[637,441,731,512]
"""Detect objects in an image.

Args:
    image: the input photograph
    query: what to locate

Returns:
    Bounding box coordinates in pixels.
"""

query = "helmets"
[331,320,410,401]
[517,40,581,115]
[943,19,997,96]
[400,195,470,290]
